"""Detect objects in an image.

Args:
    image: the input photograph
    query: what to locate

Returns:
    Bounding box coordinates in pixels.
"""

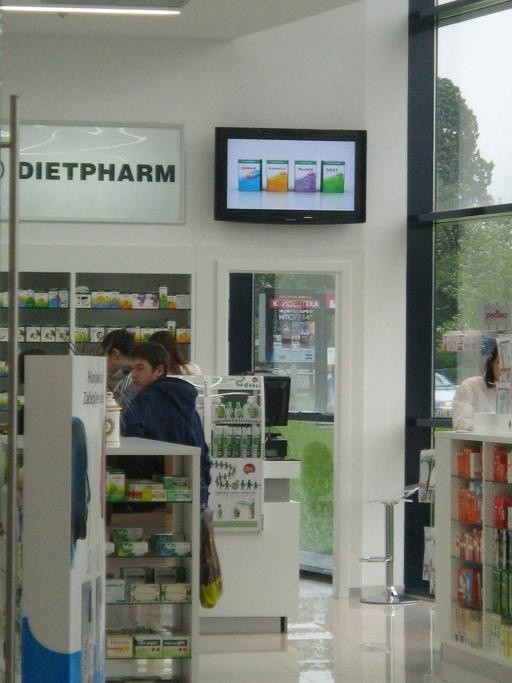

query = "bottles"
[227,396,258,419]
[212,425,260,459]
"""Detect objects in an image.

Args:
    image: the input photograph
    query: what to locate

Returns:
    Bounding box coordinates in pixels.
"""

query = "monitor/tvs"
[264,377,290,426]
[215,127,368,224]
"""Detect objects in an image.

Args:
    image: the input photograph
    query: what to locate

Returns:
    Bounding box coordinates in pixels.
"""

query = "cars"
[435,372,457,419]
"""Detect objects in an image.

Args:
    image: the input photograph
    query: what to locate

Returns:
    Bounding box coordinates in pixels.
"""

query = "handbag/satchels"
[200,509,224,608]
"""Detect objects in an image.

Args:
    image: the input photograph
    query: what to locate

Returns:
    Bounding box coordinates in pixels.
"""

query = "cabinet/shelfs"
[433,433,512,681]
[97,439,197,683]
[1,269,198,451]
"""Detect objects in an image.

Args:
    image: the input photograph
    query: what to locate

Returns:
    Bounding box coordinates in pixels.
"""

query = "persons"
[123,343,224,608]
[145,331,214,444]
[17,349,48,432]
[450,346,505,429]
[102,330,139,413]
[71,417,90,561]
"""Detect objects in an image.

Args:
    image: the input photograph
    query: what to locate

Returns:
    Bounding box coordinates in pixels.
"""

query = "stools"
[358,481,423,606]
